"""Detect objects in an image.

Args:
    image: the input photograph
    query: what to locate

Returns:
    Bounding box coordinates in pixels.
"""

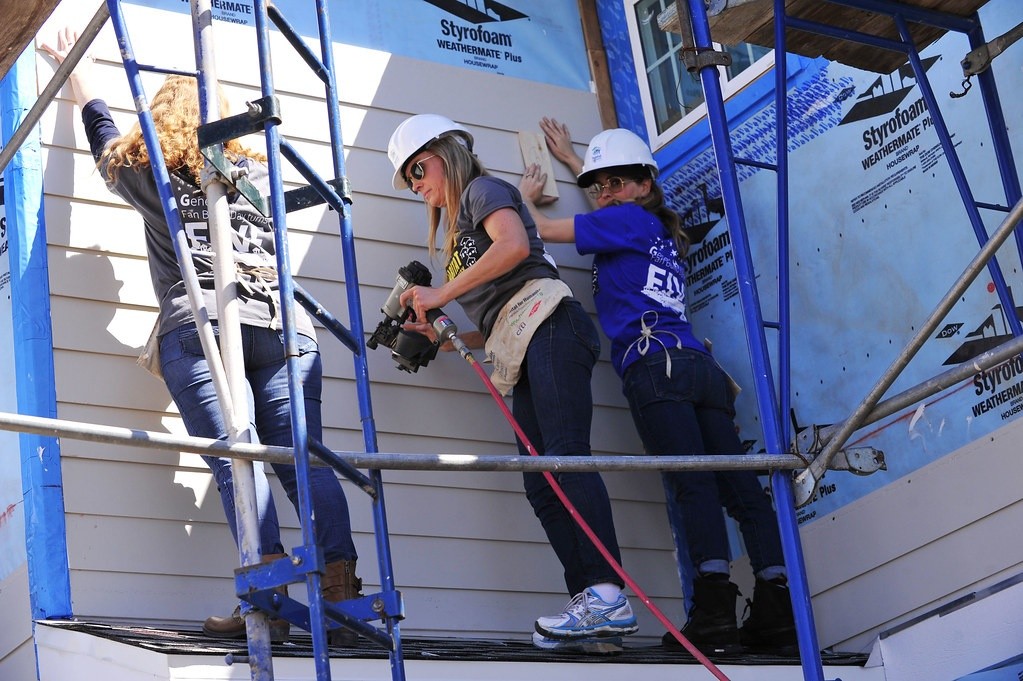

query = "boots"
[321,559,362,647]
[737,572,799,657]
[203,553,289,642]
[661,576,742,656]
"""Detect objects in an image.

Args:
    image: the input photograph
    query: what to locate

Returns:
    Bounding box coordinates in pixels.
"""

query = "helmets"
[577,128,659,188]
[387,114,474,192]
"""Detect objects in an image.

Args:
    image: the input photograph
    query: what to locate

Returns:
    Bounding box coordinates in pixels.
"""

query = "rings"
[69,42,74,45]
[526,173,534,177]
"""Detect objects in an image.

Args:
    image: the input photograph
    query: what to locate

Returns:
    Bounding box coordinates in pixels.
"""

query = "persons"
[386,112,641,647]
[40,26,367,633]
[518,115,800,656]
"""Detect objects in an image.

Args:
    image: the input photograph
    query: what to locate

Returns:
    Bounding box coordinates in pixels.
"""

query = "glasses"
[587,176,642,199]
[406,154,438,187]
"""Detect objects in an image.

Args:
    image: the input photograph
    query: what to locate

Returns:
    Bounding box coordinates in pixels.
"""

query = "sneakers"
[532,632,623,654]
[534,586,640,637]
